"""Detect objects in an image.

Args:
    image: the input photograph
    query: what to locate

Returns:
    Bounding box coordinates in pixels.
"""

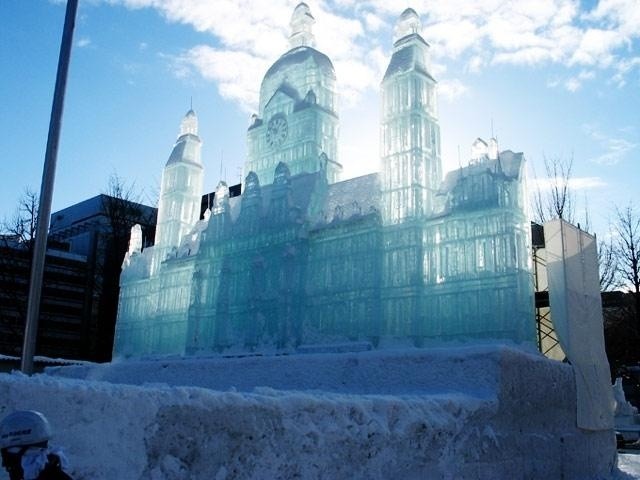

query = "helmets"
[0,410,54,449]
